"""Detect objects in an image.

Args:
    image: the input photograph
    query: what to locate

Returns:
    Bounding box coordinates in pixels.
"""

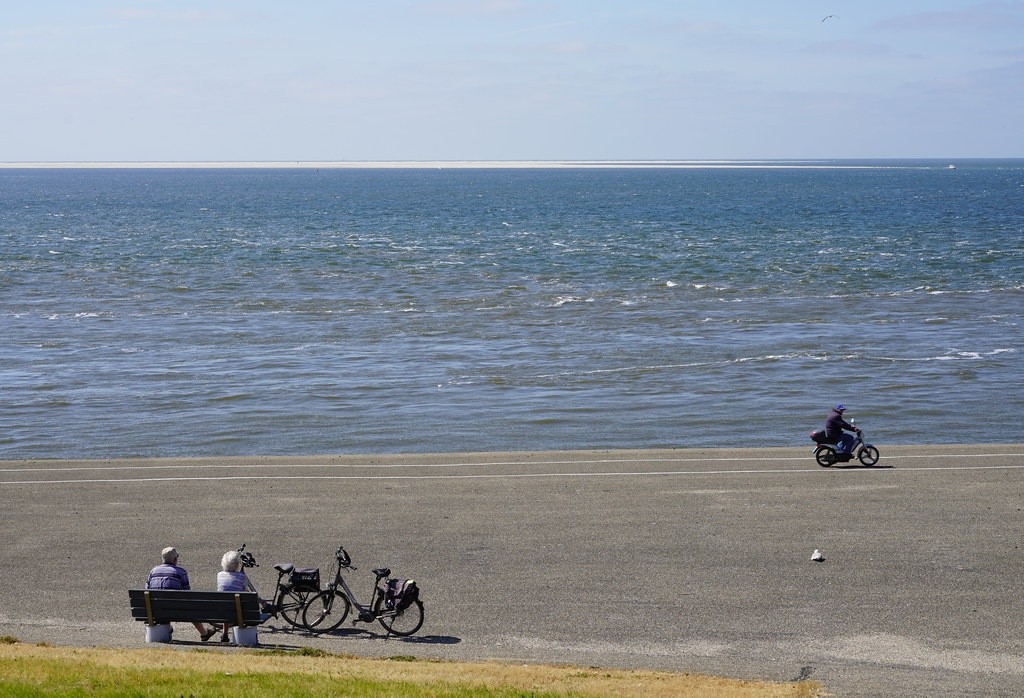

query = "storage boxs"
[810,428,825,442]
[293,567,320,592]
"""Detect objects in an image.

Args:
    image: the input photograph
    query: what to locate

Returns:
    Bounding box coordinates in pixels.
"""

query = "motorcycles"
[809,417,880,467]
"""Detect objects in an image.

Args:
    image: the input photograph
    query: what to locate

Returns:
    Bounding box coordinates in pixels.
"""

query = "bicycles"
[302,544,424,640]
[236,543,328,634]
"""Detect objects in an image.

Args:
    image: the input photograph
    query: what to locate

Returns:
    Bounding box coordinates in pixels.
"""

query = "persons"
[145,547,217,641]
[825,404,859,459]
[217,550,247,641]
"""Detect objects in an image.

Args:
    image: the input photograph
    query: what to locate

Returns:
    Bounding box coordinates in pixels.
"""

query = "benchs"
[129,590,273,647]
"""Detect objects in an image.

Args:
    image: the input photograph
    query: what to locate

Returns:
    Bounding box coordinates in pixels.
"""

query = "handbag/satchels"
[383,578,420,612]
[291,567,320,593]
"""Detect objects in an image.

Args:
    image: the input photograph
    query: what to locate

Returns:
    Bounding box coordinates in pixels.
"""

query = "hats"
[160,547,179,561]
[834,403,846,411]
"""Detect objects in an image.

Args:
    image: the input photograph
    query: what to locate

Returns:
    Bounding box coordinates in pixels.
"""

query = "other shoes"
[220,633,230,643]
[200,626,216,641]
